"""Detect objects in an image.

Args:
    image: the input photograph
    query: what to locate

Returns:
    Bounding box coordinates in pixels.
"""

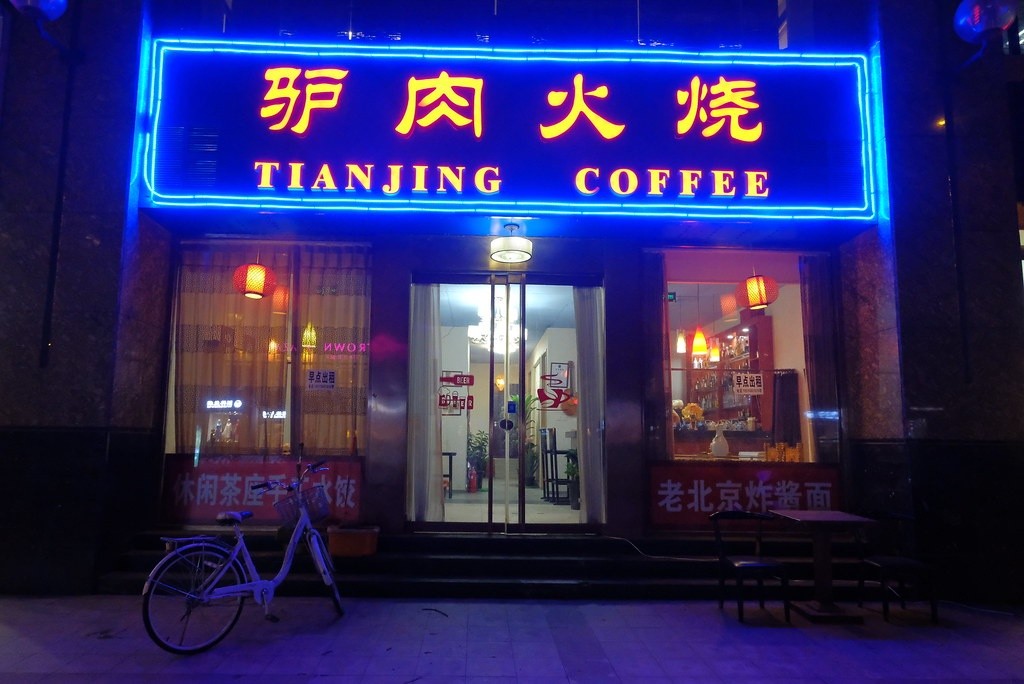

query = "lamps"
[719,294,738,322]
[734,264,779,310]
[232,249,279,301]
[691,284,709,356]
[709,293,720,363]
[272,284,290,315]
[490,224,533,264]
[676,295,687,354]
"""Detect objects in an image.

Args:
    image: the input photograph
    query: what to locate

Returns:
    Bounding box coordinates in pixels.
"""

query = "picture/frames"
[442,371,462,387]
[441,399,461,416]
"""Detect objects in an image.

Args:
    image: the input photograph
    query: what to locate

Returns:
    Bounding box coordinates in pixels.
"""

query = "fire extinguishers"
[468,464,477,493]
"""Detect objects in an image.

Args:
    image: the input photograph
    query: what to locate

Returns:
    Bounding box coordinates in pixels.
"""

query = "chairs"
[708,509,791,624]
[856,511,939,622]
[539,427,572,506]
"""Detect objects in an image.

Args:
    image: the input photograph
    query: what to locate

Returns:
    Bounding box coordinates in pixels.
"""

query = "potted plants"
[524,443,540,487]
[564,448,581,510]
[466,429,490,489]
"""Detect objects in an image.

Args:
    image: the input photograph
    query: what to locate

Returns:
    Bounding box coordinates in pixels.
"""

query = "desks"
[441,452,456,498]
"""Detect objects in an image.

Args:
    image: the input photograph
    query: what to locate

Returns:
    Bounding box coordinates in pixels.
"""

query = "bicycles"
[141,458,344,656]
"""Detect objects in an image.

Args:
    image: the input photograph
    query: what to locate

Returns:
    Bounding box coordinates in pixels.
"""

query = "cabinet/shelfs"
[684,315,774,432]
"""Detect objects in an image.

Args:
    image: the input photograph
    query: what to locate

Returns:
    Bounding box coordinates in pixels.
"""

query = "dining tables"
[768,509,869,626]
